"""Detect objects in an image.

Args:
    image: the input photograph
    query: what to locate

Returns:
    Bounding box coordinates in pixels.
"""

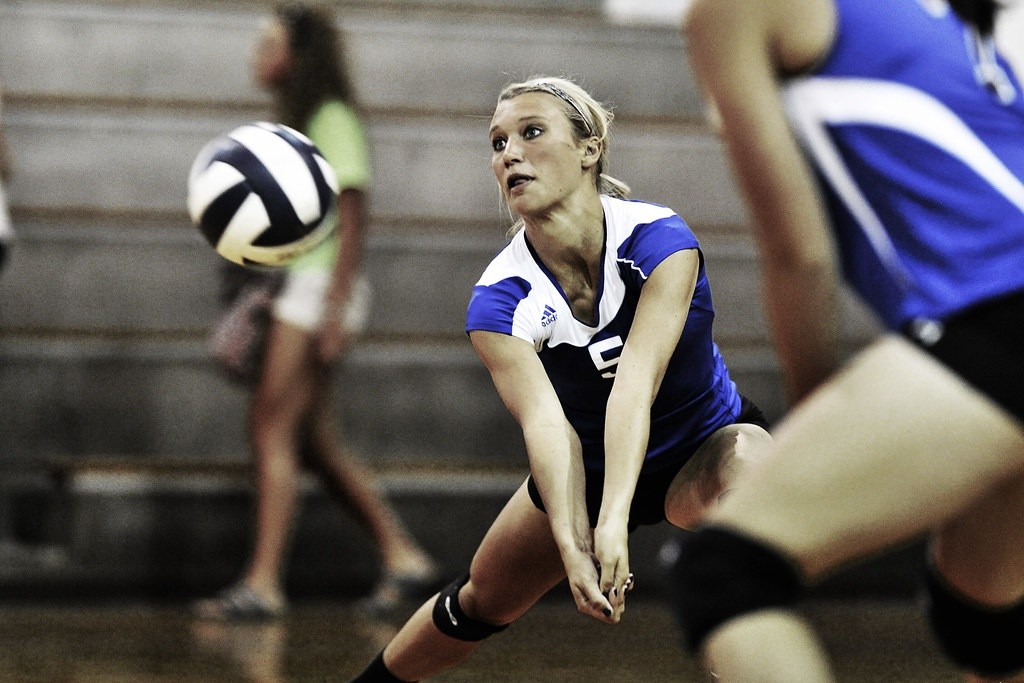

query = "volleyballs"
[187,119,338,270]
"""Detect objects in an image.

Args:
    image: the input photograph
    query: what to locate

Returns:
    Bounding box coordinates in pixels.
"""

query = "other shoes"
[196,579,291,621]
[357,565,447,615]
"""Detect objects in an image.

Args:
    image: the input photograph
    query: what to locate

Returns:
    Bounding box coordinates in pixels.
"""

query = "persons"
[672,0,1024,683]
[186,1,446,623]
[353,78,839,683]
